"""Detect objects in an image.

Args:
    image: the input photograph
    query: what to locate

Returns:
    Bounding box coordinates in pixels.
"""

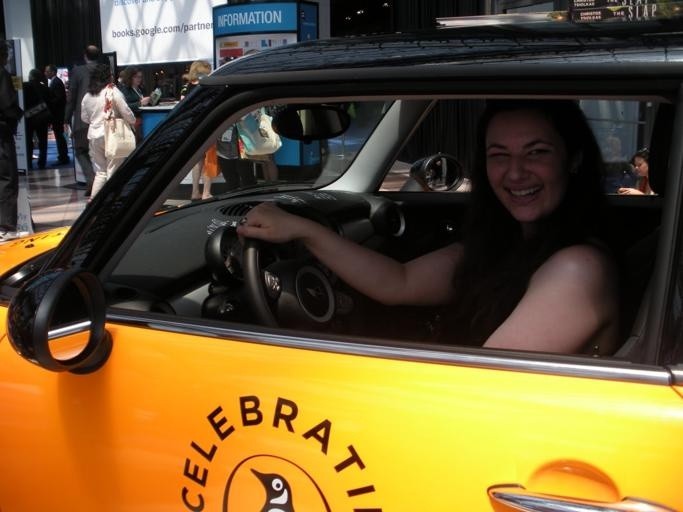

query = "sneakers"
[0,231,29,241]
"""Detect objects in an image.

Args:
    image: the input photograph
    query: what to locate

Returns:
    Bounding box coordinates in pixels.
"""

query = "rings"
[239,218,247,225]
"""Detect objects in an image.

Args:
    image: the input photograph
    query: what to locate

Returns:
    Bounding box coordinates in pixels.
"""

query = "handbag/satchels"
[237,114,283,156]
[103,118,136,158]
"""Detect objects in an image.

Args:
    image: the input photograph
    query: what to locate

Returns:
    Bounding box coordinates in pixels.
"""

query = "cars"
[1,21,683,512]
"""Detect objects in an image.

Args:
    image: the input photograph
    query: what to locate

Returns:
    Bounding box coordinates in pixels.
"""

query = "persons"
[629,160,643,189]
[618,148,657,195]
[44,65,71,165]
[124,65,150,145]
[186,60,220,201]
[80,66,137,207]
[22,70,48,170]
[235,100,621,357]
[72,45,100,197]
[0,42,29,238]
